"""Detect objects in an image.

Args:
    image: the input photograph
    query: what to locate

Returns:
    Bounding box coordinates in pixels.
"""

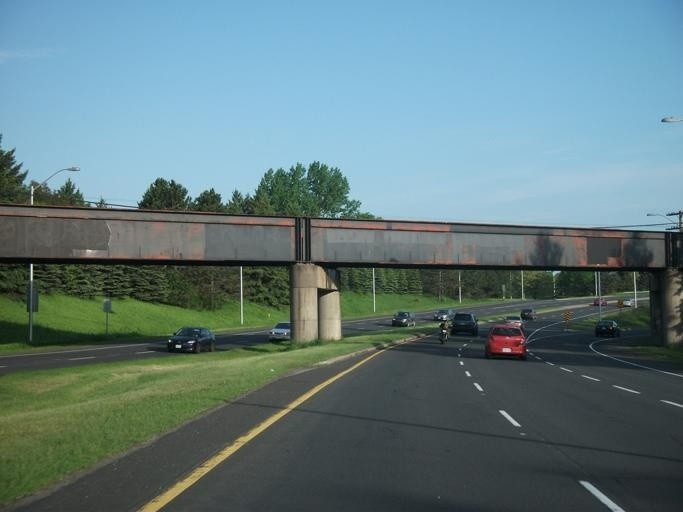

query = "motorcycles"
[439,326,451,344]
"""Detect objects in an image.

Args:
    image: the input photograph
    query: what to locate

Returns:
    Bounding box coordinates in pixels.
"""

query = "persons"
[440,320,449,339]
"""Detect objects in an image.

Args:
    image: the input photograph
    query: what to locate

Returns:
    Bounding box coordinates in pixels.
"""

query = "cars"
[451,312,478,336]
[168,326,216,353]
[268,321,291,341]
[392,311,416,327]
[594,297,607,306]
[485,323,529,360]
[623,298,640,306]
[595,320,622,338]
[504,315,523,328]
[520,307,535,320]
[434,309,457,321]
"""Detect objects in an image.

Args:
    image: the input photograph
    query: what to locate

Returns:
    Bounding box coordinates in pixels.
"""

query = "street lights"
[647,213,679,227]
[29,167,80,342]
[662,116,682,122]
